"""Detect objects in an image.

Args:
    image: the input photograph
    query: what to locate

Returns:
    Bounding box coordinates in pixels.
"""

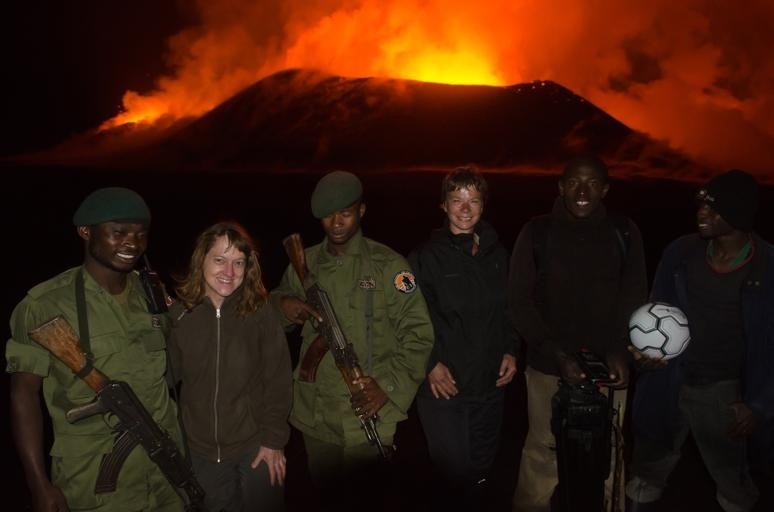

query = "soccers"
[628,301,690,361]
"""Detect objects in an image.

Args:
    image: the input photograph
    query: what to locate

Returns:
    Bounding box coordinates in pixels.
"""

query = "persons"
[625,170,774,511]
[406,164,524,512]
[163,221,295,511]
[508,154,647,511]
[7,188,193,512]
[269,169,435,511]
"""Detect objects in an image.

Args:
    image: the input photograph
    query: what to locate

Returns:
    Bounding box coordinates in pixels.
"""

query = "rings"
[741,422,747,427]
[743,430,747,435]
[279,461,284,467]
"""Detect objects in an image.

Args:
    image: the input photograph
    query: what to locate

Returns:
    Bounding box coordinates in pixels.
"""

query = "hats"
[71,187,150,227]
[697,168,766,235]
[310,170,363,219]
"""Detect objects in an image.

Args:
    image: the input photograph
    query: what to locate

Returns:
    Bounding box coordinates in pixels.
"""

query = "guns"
[27,314,204,512]
[282,234,386,459]
